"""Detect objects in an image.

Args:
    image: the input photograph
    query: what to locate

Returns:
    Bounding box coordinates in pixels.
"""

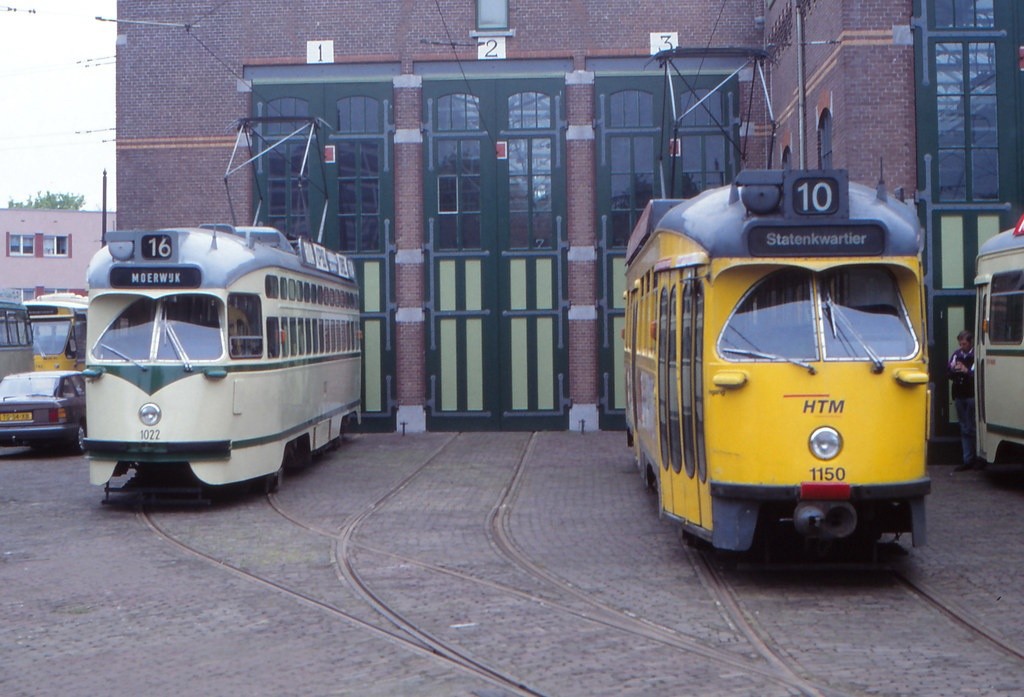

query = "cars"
[0,368,88,456]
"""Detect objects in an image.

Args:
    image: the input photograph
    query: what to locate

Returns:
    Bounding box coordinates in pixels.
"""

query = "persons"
[945,330,976,472]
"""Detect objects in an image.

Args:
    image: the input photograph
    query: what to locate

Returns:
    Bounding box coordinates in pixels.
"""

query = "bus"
[75,222,367,494]
[969,213,1024,475]
[621,160,937,570]
[0,298,44,384]
[20,290,88,372]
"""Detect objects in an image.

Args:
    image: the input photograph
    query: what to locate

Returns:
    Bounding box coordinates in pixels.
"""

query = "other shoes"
[972,459,986,469]
[954,463,972,472]
